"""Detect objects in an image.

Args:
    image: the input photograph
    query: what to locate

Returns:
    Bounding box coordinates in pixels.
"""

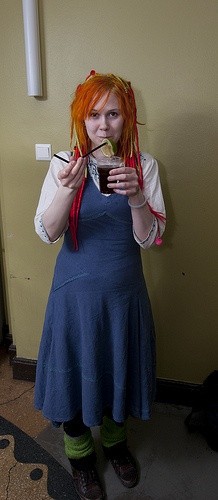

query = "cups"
[97,156,122,194]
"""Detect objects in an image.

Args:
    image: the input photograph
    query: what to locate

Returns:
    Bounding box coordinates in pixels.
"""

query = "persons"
[34,70,167,500]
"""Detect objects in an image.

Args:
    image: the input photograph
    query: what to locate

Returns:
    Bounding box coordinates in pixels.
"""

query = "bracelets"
[128,195,148,209]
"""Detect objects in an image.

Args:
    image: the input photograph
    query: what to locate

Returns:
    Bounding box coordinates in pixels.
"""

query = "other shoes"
[102,442,138,488]
[68,452,105,500]
